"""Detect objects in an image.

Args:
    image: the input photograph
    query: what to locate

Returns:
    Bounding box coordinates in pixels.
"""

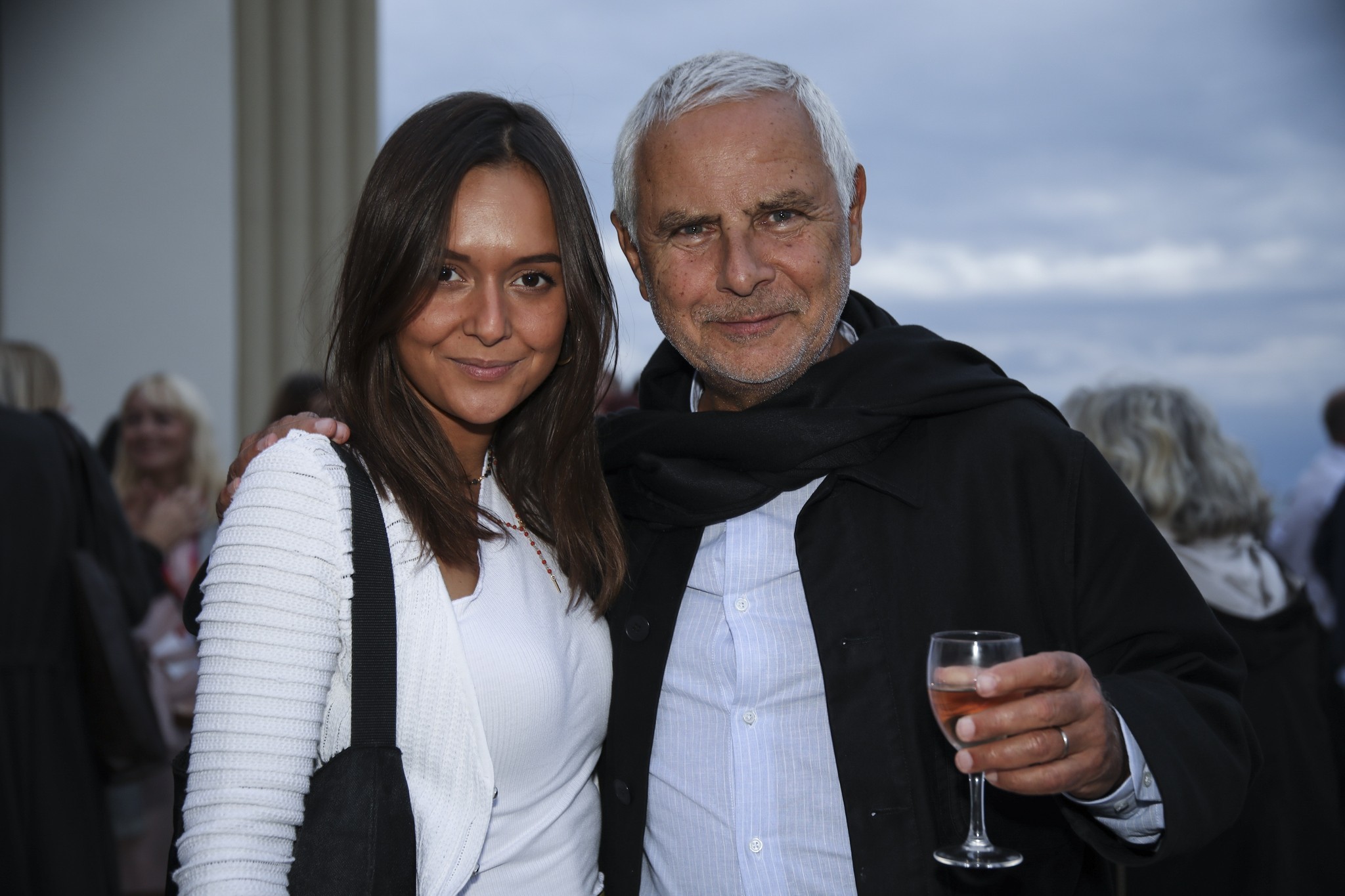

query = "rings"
[1053,724,1069,760]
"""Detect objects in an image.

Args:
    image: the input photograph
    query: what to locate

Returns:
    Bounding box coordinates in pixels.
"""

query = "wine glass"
[927,630,1023,868]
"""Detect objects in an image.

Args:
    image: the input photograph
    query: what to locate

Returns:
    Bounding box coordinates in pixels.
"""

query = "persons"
[213,53,1251,895]
[0,401,161,894]
[1059,380,1345,892]
[1262,385,1345,684]
[167,88,615,895]
[268,371,334,416]
[2,341,72,415]
[93,375,201,894]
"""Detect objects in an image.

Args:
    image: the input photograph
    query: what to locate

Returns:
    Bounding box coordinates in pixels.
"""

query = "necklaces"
[461,444,563,593]
